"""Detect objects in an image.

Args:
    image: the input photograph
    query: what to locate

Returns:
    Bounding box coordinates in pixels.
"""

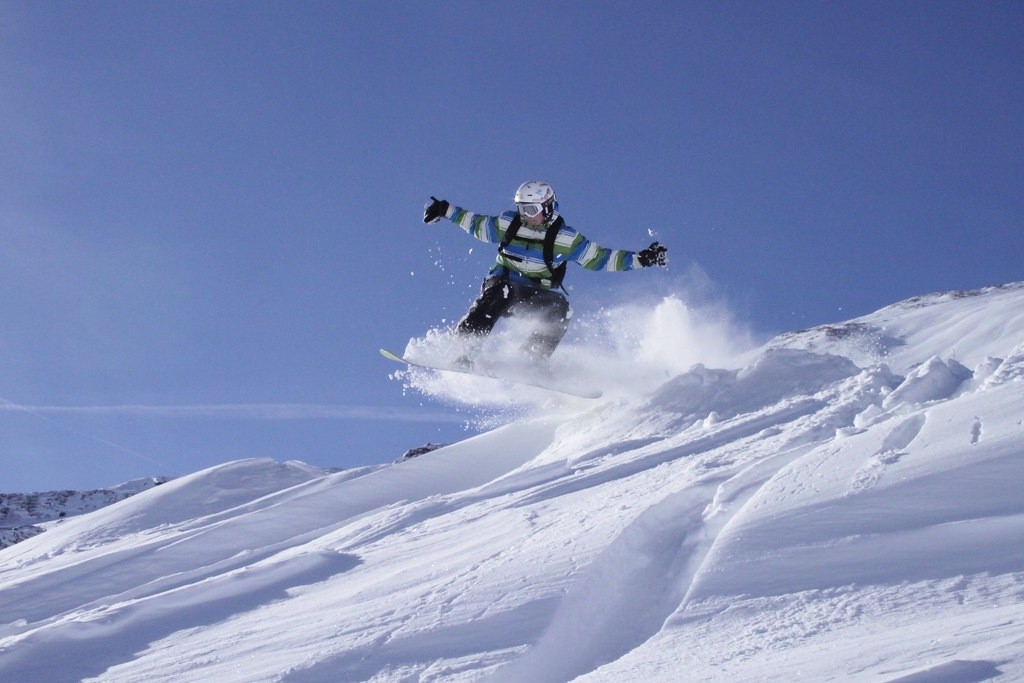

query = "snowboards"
[380,348,603,400]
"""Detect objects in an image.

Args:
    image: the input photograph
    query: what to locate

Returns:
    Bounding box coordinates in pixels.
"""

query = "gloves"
[422,194,449,224]
[637,241,668,267]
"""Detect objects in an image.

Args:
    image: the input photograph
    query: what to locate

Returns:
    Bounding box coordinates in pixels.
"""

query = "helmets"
[514,180,553,218]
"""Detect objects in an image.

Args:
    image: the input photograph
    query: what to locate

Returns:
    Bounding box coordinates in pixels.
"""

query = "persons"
[421,180,671,385]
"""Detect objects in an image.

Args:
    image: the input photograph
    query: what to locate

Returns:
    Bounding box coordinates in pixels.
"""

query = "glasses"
[516,201,545,218]
